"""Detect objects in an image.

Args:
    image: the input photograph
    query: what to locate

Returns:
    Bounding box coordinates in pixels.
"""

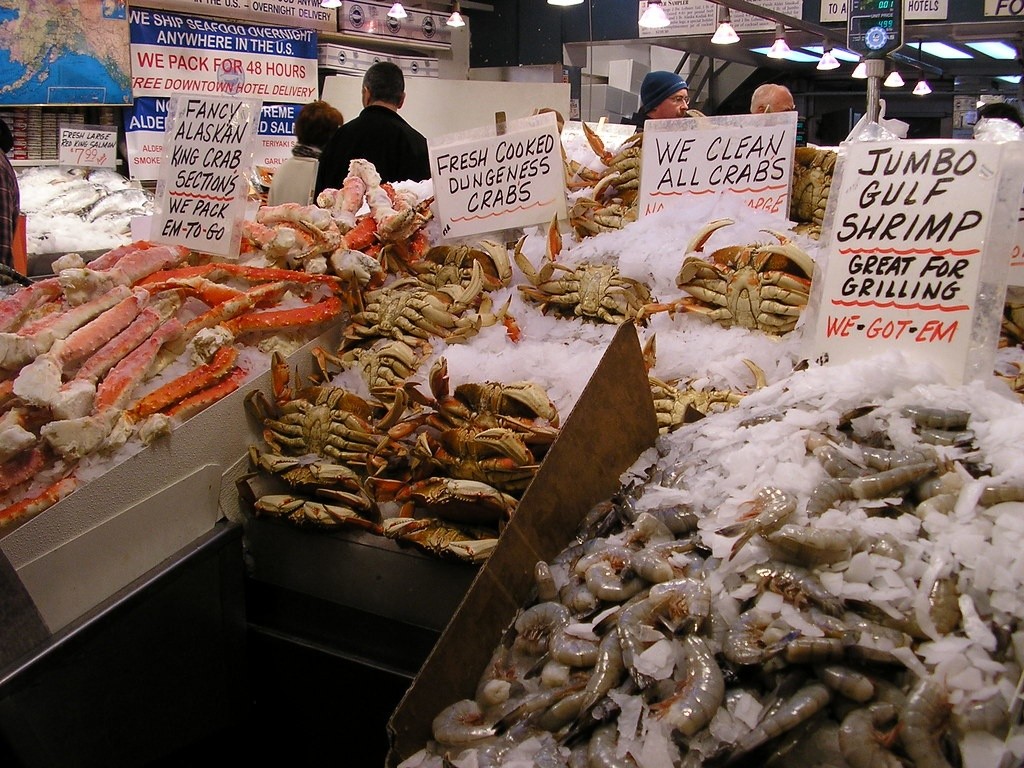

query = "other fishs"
[11,163,156,237]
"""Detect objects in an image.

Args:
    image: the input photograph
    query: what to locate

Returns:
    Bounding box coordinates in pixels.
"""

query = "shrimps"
[428,399,1024,768]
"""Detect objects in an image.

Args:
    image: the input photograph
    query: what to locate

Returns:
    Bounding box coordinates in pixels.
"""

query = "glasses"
[667,96,691,106]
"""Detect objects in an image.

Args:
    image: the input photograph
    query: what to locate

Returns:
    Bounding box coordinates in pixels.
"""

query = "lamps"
[816,38,840,70]
[912,71,932,95]
[767,24,792,58]
[852,57,868,78]
[711,7,740,44]
[387,0,407,18]
[884,62,905,87]
[321,0,342,7]
[446,0,465,26]
[638,0,670,28]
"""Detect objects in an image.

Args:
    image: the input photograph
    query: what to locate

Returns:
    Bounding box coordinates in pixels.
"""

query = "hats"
[641,71,688,113]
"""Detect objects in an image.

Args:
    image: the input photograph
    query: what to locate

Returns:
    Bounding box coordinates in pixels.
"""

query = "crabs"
[1,156,435,539]
[241,119,840,564]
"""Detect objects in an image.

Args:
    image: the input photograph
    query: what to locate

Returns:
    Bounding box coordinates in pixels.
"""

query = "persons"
[749,84,795,114]
[314,62,431,206]
[267,99,343,207]
[0,118,21,270]
[975,102,1024,128]
[619,71,690,129]
